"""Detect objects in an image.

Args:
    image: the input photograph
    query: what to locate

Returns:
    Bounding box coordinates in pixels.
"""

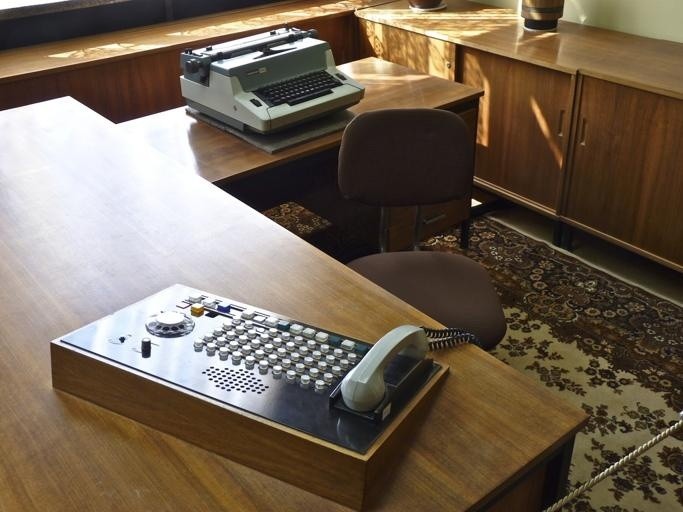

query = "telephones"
[49,283,484,512]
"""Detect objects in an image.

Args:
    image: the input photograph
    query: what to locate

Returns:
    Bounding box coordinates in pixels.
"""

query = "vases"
[408,0,448,12]
[521,0,565,33]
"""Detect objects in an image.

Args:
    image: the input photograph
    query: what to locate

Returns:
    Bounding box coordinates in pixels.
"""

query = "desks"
[116,56,485,256]
[0,93,590,512]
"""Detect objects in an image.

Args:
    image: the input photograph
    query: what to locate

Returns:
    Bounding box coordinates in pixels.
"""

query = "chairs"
[336,108,508,351]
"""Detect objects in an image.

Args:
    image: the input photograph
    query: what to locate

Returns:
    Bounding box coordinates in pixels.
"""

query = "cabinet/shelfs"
[0,0,403,124]
[558,69,683,274]
[353,16,456,82]
[455,39,579,246]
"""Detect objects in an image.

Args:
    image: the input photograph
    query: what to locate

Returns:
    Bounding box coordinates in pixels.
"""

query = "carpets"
[260,200,683,512]
